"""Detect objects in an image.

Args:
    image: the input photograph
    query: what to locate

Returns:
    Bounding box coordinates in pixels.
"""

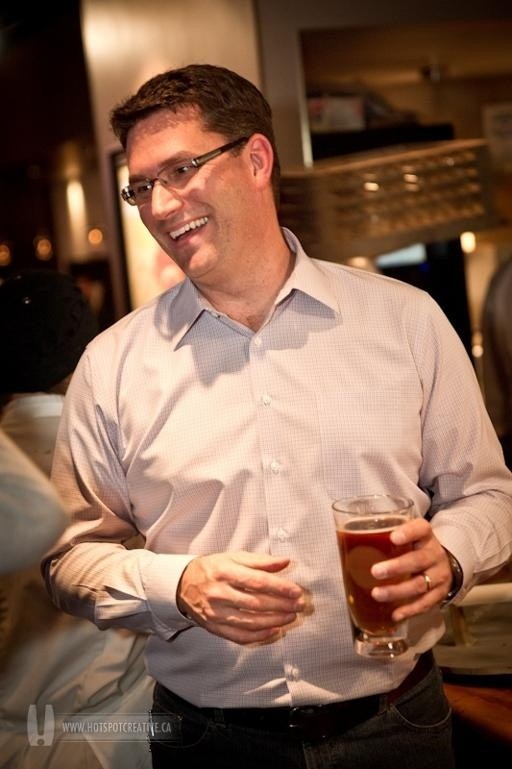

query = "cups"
[328,491,417,663]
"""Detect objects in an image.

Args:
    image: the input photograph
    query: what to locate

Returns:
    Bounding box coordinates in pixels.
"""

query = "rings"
[418,571,431,591]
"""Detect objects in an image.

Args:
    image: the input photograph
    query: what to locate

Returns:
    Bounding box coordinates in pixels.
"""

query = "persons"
[38,56,512,767]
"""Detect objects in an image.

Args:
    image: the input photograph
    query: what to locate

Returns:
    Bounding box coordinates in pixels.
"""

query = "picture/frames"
[107,144,200,315]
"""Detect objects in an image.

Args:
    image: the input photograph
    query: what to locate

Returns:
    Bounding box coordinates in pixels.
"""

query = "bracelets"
[438,544,464,609]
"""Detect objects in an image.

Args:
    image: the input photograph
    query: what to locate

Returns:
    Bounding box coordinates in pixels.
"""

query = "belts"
[156,648,435,743]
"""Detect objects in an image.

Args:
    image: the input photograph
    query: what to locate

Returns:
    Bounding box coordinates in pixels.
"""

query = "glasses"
[119,134,260,206]
